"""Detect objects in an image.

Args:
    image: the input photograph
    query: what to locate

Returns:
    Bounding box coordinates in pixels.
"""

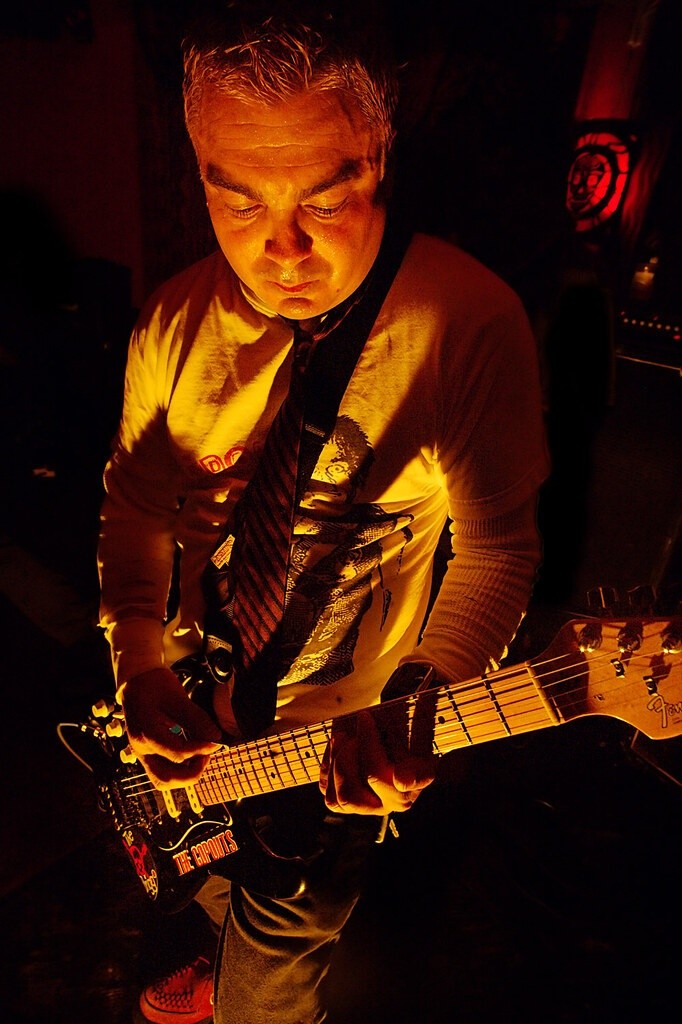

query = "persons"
[97,0,548,1024]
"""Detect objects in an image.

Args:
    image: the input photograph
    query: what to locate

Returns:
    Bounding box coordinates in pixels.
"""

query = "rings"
[165,724,185,742]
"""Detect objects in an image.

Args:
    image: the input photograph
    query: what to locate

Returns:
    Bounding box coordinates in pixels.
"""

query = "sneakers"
[140,956,215,1024]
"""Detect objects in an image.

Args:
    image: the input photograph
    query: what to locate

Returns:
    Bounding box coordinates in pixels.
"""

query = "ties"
[222,288,370,672]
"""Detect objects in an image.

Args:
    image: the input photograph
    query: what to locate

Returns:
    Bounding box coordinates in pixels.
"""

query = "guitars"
[57,575,681,905]
